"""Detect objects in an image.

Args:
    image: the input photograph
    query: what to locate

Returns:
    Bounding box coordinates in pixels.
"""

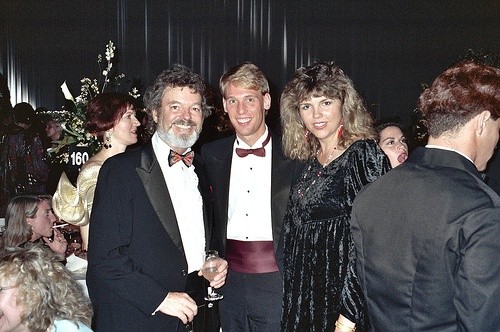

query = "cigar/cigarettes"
[52,223,69,228]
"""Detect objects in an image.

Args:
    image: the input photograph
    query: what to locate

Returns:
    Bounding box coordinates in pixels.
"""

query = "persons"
[373,117,408,169]
[0,102,96,332]
[278,62,393,332]
[200,62,305,332]
[135,104,153,144]
[85,64,228,332]
[349,62,500,332]
[76,89,142,254]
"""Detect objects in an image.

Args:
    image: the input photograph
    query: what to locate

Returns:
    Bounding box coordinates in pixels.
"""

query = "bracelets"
[335,320,356,332]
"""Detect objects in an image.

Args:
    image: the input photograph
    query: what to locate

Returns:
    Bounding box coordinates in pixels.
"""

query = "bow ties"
[168,149,194,168]
[236,147,265,158]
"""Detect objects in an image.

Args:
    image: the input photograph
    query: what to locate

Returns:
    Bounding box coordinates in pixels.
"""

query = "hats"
[14,102,34,125]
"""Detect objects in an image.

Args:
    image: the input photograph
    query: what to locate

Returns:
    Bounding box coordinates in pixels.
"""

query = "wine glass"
[71,237,82,256]
[202,250,223,301]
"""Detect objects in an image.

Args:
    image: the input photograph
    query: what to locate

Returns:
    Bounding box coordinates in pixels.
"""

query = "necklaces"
[297,139,345,197]
